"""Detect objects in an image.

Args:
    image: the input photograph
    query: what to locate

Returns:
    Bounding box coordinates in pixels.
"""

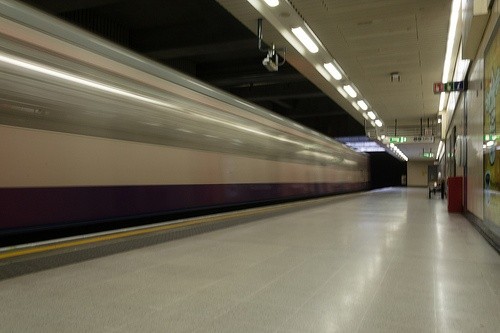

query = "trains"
[0,1,365,247]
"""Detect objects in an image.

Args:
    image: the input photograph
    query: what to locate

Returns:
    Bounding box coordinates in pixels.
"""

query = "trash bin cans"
[445,176,462,214]
